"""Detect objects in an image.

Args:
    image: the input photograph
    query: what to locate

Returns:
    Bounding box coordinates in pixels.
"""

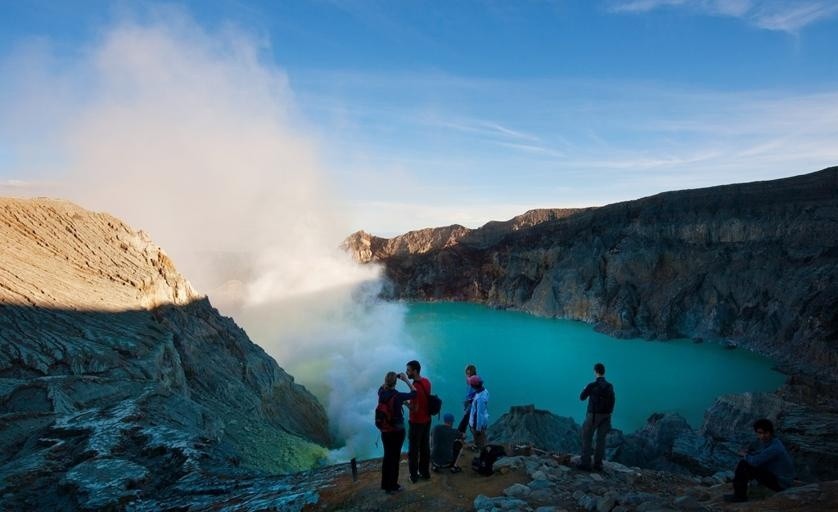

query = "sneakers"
[381,465,463,493]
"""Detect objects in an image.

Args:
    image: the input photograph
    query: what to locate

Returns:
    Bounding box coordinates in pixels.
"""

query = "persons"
[577,363,615,466]
[724,419,794,503]
[375,360,489,494]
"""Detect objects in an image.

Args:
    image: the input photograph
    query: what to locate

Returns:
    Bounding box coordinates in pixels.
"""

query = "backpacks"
[375,393,400,433]
[425,393,442,416]
[594,383,614,416]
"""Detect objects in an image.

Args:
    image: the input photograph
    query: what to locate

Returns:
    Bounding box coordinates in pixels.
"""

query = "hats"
[466,376,482,386]
[444,413,455,422]
